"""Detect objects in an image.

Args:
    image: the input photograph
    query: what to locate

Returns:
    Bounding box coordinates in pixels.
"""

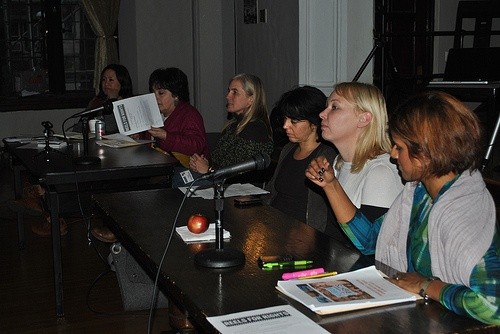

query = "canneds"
[95,121,105,139]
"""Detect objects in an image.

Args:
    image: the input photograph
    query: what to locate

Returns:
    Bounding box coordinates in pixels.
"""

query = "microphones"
[198,152,271,180]
[71,103,113,118]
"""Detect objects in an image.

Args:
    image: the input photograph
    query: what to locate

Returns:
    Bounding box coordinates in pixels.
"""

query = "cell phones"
[258,255,295,264]
[234,198,263,204]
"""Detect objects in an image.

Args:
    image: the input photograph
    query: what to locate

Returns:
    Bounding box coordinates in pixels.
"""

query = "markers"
[281,267,325,279]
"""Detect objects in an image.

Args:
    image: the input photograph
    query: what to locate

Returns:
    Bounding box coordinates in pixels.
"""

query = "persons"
[31,64,144,236]
[261,86,339,232]
[91,67,210,243]
[319,82,406,258]
[189,73,274,189]
[304,90,500,327]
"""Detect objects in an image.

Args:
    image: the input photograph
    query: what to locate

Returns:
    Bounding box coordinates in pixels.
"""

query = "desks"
[3,131,180,318]
[89,188,500,334]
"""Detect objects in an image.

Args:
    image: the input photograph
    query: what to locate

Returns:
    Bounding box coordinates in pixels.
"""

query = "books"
[36,138,68,149]
[275,265,424,315]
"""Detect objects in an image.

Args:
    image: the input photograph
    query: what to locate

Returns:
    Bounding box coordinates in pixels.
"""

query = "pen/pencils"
[297,271,337,282]
[260,260,313,267]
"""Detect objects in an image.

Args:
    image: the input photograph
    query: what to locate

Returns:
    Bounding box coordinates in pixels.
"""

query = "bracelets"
[419,276,441,302]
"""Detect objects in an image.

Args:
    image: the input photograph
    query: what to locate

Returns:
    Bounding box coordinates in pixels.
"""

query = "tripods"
[34,121,64,162]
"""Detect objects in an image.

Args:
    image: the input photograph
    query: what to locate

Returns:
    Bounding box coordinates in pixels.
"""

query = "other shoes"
[8,197,42,217]
[32,218,67,236]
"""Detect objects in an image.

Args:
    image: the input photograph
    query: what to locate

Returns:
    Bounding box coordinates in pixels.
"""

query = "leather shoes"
[91,226,118,243]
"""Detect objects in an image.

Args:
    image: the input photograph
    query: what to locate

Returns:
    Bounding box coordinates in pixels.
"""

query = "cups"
[89,120,100,133]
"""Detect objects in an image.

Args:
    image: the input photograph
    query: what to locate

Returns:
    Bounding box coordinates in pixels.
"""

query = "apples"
[189,243,209,253]
[187,214,208,234]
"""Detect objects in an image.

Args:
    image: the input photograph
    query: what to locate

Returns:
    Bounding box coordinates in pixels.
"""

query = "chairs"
[424,47,500,174]
